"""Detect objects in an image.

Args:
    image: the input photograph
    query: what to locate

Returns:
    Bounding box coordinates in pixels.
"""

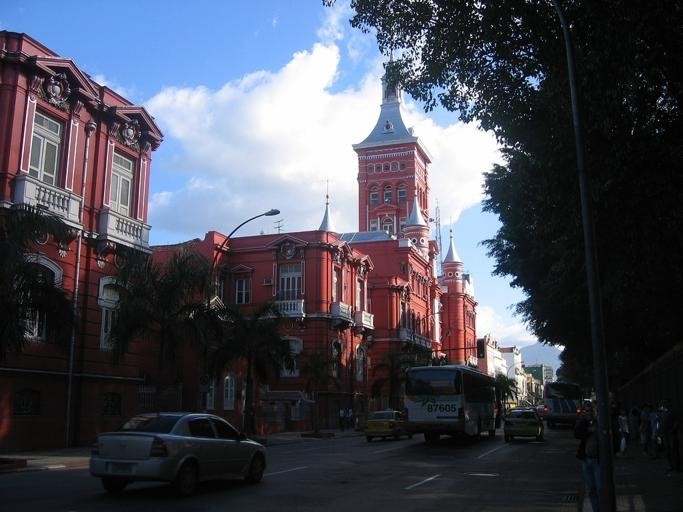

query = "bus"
[542,382,584,429]
[404,364,502,445]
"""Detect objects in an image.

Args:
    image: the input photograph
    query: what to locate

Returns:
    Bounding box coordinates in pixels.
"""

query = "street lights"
[202,207,280,415]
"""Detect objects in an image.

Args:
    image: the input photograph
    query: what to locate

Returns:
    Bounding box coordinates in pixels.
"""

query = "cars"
[89,410,268,497]
[364,409,413,441]
[503,407,545,442]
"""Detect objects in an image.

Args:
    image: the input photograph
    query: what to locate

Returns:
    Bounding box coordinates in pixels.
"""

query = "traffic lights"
[476,340,486,358]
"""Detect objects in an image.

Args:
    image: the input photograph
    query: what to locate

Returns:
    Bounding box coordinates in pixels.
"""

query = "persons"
[575,397,682,512]
